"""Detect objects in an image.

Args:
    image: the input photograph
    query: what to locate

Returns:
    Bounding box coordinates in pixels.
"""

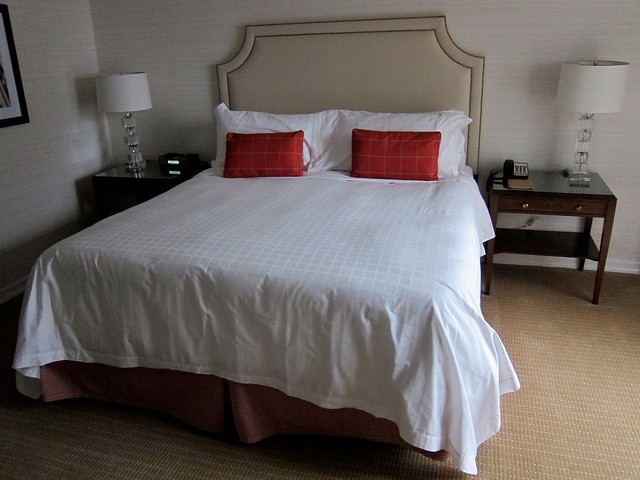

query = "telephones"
[503,159,529,179]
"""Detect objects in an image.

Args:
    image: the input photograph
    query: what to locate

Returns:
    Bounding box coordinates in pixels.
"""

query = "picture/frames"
[0,3,32,127]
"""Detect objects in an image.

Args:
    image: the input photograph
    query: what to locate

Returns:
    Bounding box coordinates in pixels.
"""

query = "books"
[508,177,532,191]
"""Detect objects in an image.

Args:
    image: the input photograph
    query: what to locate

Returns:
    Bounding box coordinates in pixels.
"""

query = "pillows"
[223,129,304,178]
[214,103,331,174]
[347,129,442,182]
[333,111,470,181]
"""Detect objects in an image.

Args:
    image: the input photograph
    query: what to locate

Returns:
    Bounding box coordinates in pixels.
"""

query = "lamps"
[555,59,630,189]
[96,70,154,171]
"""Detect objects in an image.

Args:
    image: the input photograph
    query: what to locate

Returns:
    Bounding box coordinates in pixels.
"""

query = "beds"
[12,15,523,476]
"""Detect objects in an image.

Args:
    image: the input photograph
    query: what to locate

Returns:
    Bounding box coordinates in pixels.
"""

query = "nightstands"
[78,163,199,225]
[484,170,620,305]
